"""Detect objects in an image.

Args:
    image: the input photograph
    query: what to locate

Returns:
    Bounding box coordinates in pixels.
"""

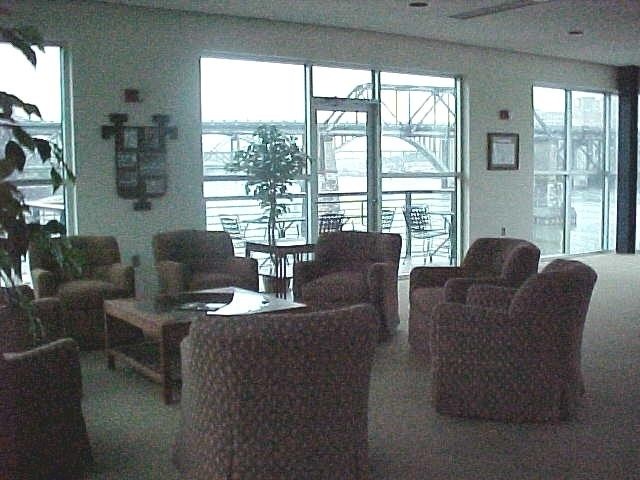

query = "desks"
[246,242,315,299]
[242,218,305,269]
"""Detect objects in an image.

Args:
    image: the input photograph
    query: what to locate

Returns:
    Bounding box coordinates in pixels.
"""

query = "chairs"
[319,214,350,234]
[218,215,248,249]
[382,208,396,232]
[402,205,456,265]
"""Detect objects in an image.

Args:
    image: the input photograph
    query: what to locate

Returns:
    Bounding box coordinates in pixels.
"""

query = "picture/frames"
[488,133,519,170]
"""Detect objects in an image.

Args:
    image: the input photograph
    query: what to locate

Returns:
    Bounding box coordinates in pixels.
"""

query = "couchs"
[153,231,258,299]
[1,285,35,306]
[431,259,597,424]
[29,236,134,349]
[0,299,91,479]
[293,231,402,343]
[175,303,376,480]
[409,238,541,356]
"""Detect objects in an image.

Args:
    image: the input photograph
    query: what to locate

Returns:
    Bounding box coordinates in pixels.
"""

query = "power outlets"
[501,227,506,236]
[133,255,141,267]
[500,110,509,120]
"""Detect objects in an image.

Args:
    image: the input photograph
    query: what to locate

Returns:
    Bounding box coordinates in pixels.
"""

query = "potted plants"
[224,126,316,294]
